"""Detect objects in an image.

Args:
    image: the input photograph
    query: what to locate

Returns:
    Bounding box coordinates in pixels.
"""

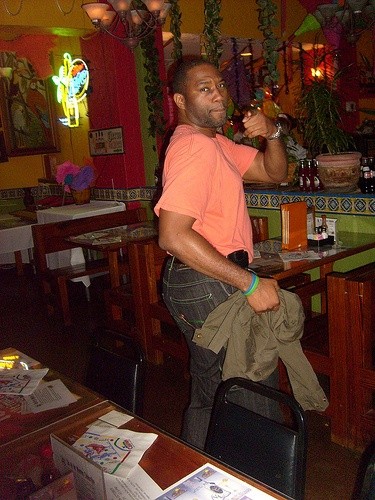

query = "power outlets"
[346,102,356,112]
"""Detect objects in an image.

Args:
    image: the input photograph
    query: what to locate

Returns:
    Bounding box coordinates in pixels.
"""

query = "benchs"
[31,207,375,451]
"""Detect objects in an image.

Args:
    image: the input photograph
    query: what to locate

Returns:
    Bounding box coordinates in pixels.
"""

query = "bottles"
[318,215,328,234]
[357,157,375,195]
[22,187,34,210]
[299,159,324,193]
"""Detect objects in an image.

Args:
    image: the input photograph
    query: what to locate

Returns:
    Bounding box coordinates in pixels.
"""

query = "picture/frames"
[88,126,124,156]
[0,51,62,156]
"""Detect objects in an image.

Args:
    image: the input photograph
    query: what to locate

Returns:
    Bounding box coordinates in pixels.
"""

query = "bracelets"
[268,122,282,140]
[244,274,260,296]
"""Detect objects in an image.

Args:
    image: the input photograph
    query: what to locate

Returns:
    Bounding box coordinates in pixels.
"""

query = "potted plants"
[292,62,362,189]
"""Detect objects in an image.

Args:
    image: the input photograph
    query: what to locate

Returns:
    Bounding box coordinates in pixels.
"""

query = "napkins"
[278,250,321,261]
[72,425,158,480]
[0,368,50,396]
[92,236,122,245]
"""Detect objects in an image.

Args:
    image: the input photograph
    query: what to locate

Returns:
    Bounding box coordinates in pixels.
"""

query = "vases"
[23,187,34,207]
[70,186,90,206]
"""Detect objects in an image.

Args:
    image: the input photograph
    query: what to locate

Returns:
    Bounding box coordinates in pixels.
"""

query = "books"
[280,202,307,250]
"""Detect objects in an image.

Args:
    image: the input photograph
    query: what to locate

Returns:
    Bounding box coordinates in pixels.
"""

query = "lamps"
[81,0,172,54]
[317,0,375,48]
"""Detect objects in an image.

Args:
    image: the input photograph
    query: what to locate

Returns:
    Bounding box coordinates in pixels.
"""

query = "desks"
[249,231,375,314]
[9,208,37,276]
[66,219,159,345]
[36,200,127,302]
[0,345,108,447]
[0,399,297,500]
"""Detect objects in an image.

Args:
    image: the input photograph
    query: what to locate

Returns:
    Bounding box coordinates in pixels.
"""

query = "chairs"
[85,326,146,416]
[202,377,308,500]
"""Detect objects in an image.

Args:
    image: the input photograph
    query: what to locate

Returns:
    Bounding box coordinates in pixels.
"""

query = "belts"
[168,256,181,264]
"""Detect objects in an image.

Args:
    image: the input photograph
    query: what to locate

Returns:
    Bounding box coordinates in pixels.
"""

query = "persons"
[154,59,289,450]
